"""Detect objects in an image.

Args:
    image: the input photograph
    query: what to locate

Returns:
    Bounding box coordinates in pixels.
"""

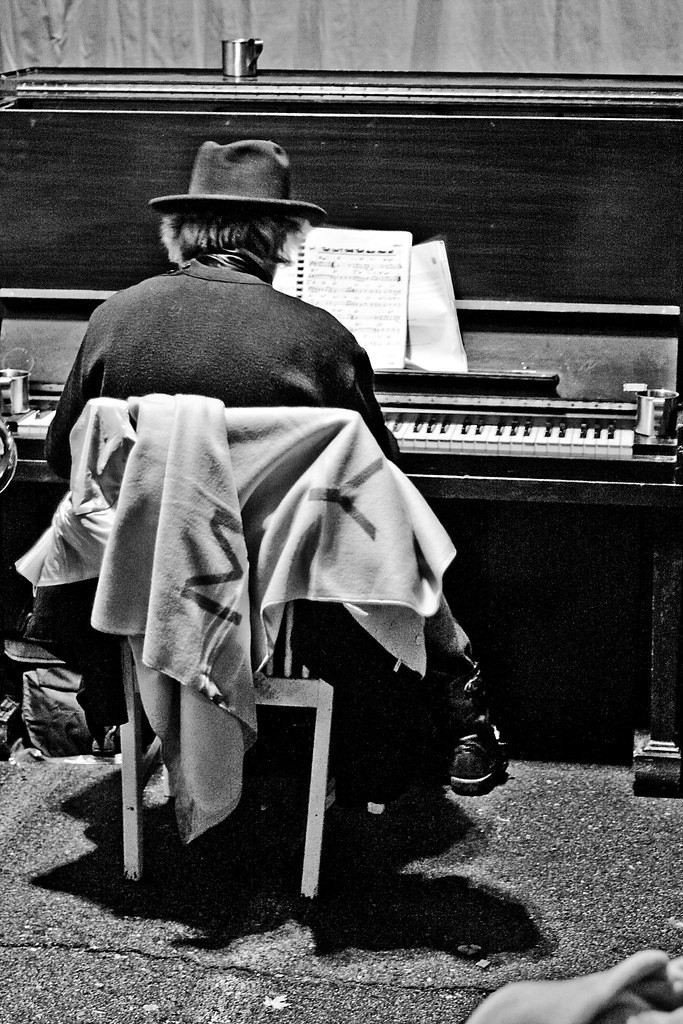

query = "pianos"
[0,64,682,802]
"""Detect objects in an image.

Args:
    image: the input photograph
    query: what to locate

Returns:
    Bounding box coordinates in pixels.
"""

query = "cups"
[219,37,264,78]
[632,388,681,439]
[0,368,32,416]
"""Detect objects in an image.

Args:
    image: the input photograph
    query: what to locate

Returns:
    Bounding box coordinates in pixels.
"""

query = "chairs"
[78,397,358,900]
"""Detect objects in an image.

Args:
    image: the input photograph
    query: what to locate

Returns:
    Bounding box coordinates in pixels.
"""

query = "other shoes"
[449,722,512,793]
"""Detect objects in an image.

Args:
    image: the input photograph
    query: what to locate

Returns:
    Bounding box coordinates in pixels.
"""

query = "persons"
[45,140,511,798]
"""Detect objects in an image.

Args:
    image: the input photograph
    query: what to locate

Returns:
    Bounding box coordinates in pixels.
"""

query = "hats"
[149,140,327,228]
